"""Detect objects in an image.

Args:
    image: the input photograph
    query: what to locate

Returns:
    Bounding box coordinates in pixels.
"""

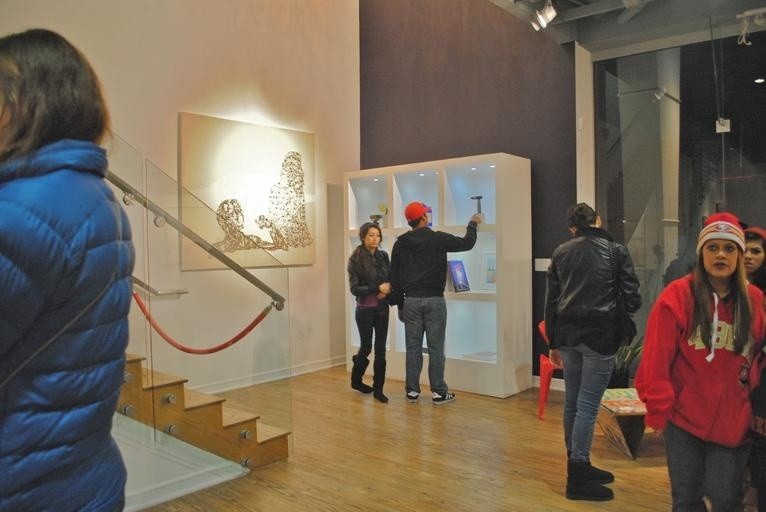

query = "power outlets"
[715,119,732,133]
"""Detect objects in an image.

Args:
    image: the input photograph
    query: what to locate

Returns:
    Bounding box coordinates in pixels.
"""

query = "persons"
[347,222,391,403]
[392,202,483,405]
[634,211,766,512]
[741,226,766,512]
[1,27,135,511]
[543,203,643,502]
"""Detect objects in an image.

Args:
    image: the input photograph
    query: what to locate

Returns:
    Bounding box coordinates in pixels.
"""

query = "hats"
[697,212,747,255]
[745,228,766,241]
[406,203,432,222]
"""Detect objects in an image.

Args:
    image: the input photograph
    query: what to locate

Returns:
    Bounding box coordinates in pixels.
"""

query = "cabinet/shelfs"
[341,153,535,400]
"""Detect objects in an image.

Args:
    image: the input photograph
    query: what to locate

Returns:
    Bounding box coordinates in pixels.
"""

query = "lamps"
[527,1,559,32]
[648,86,668,103]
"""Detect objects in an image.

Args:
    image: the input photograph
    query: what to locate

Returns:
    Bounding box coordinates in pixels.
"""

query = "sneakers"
[406,391,421,403]
[433,392,456,405]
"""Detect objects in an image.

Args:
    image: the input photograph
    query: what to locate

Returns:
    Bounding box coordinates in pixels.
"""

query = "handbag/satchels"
[607,239,638,346]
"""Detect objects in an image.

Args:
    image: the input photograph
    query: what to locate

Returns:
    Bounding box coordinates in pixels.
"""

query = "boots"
[351,355,375,394]
[373,359,390,403]
[566,459,613,502]
[569,453,616,485]
